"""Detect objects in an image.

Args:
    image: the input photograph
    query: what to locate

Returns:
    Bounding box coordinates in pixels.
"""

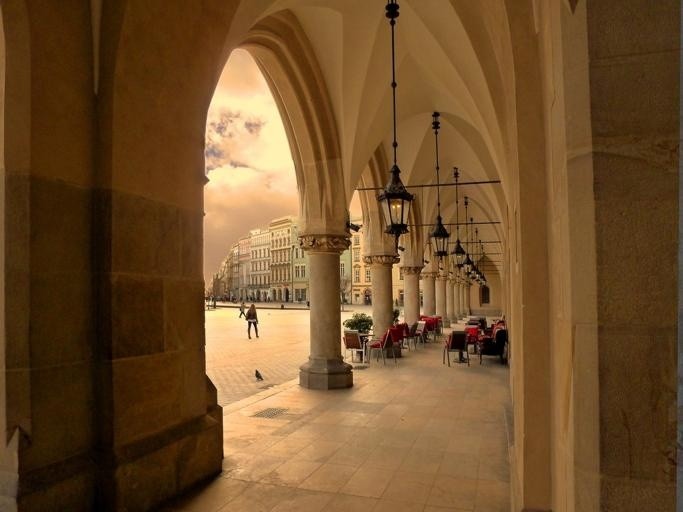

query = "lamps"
[375,0,477,288]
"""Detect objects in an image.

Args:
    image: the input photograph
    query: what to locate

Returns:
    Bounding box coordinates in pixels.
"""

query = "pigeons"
[256,370,263,381]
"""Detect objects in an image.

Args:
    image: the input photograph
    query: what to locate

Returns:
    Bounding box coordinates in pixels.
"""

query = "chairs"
[342,315,441,367]
[442,317,505,367]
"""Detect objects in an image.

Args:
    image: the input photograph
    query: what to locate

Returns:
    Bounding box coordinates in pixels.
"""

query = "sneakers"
[248,335,259,339]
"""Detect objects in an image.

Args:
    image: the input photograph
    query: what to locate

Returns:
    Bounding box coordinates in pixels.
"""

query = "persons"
[239,302,245,318]
[245,304,259,339]
